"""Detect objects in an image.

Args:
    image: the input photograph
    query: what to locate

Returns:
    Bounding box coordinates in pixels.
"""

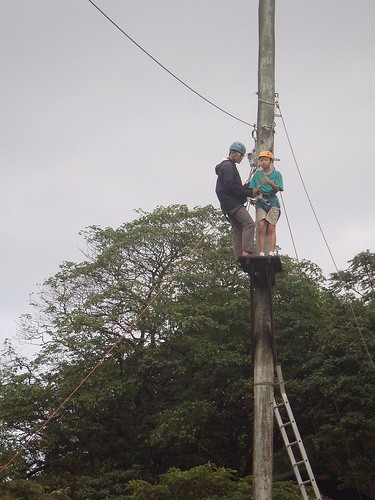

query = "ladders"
[273,364,323,500]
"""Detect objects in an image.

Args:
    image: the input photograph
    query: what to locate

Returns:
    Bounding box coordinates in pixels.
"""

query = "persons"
[215,141,258,267]
[250,150,283,257]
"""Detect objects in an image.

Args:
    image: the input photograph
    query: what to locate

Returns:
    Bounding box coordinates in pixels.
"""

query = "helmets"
[230,142,246,154]
[259,150,273,159]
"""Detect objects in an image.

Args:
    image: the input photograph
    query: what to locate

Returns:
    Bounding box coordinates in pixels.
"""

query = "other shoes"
[269,251,275,256]
[260,252,265,256]
